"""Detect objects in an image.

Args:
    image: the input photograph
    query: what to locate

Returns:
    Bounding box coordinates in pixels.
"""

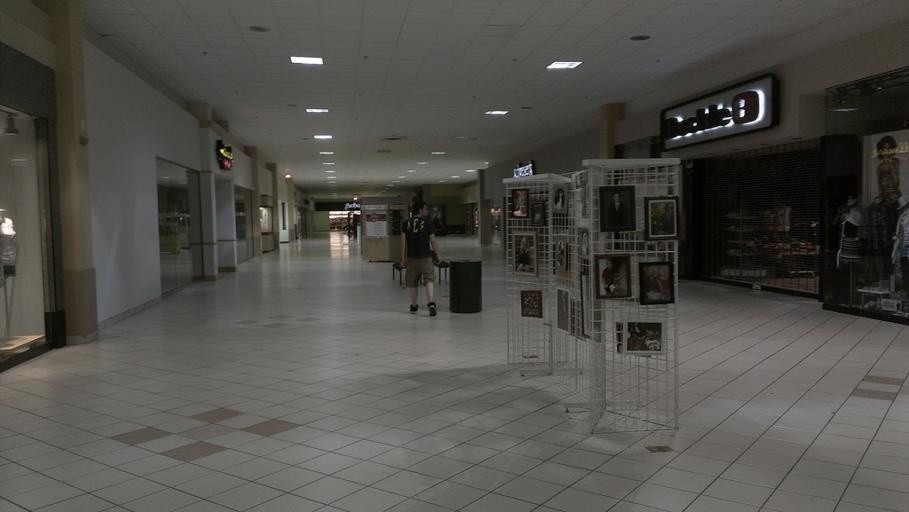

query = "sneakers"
[409,303,419,313]
[428,302,436,315]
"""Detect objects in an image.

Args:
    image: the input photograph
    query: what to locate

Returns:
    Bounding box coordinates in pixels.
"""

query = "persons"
[863,198,891,265]
[0,209,18,350]
[602,260,617,293]
[400,200,444,316]
[837,197,866,275]
[579,232,589,254]
[607,193,626,225]
[889,196,909,290]
[554,188,565,208]
[871,135,902,217]
[514,192,528,218]
[628,323,659,351]
[556,241,563,255]
[653,204,672,233]
[515,237,532,271]
[644,267,666,300]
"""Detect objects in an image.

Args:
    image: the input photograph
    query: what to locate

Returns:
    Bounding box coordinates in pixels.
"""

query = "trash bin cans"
[449,258,481,313]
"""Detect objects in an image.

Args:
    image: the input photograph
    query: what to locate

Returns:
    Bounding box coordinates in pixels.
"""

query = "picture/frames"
[512,183,681,358]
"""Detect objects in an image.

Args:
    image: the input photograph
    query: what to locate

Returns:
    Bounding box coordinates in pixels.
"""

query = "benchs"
[434,260,449,286]
[392,263,407,286]
